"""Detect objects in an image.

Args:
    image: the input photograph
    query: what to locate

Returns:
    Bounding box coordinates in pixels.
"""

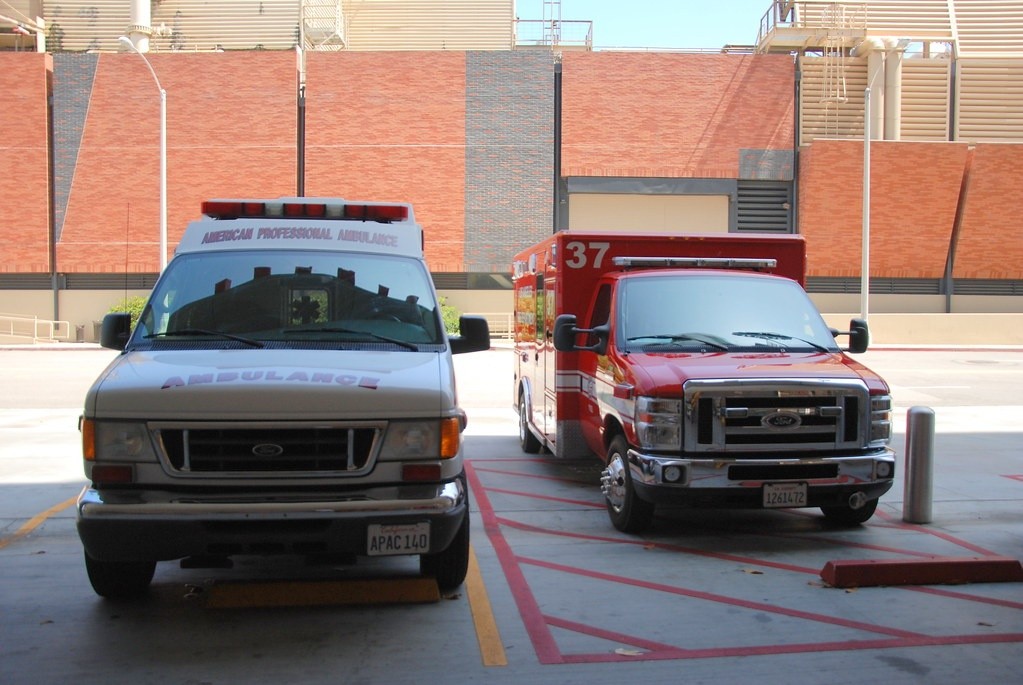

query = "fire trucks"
[510,230,896,533]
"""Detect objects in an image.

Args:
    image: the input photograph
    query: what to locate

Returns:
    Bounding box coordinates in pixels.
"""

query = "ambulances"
[77,198,489,605]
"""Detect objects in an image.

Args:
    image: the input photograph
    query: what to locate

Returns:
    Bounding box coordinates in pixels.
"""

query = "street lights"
[861,38,911,322]
[120,35,168,283]
